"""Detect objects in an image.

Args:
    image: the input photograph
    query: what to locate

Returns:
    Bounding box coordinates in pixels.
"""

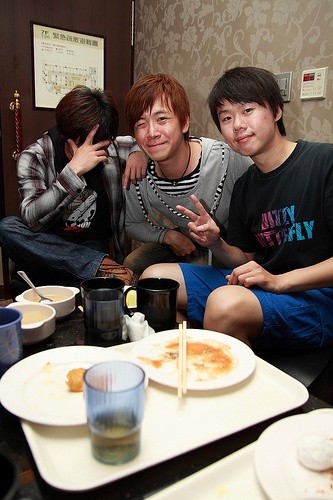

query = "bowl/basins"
[5,302,57,344]
[15,285,80,320]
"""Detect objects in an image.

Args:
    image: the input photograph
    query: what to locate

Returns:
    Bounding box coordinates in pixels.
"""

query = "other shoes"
[98,258,139,286]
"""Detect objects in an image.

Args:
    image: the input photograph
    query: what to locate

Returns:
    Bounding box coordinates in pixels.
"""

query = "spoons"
[17,271,53,302]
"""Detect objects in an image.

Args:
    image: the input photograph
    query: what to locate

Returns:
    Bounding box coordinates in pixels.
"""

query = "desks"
[0,302,333,500]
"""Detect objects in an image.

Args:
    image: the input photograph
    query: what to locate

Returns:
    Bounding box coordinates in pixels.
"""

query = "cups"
[0,307,23,365]
[123,278,179,332]
[83,360,146,465]
[84,288,123,347]
[80,277,125,306]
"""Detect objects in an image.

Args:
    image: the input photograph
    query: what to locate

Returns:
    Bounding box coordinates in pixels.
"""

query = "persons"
[122,73,254,274]
[138,66,333,348]
[0,84,150,287]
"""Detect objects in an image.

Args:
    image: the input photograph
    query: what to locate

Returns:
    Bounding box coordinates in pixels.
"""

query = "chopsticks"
[178,321,186,398]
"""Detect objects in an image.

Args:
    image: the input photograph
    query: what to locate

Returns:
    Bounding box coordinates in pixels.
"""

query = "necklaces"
[158,143,191,186]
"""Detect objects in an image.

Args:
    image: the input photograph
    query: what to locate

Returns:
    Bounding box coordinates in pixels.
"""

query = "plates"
[131,329,257,391]
[0,346,149,427]
[123,285,136,308]
[254,412,333,500]
